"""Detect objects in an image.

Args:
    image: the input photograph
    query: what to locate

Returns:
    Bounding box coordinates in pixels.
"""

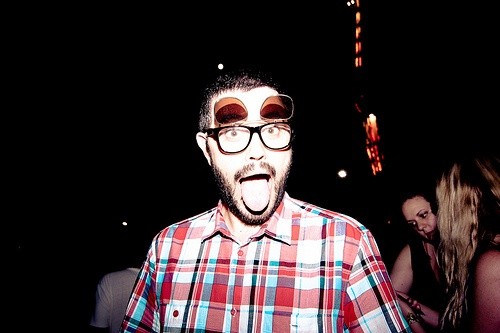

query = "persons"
[119,67,415,333]
[89,231,148,333]
[387,180,461,333]
[392,152,500,333]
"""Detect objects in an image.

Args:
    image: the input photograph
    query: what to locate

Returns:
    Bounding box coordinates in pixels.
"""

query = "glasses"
[204,94,296,153]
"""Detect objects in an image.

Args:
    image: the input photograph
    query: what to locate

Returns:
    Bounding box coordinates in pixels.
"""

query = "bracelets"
[406,311,419,325]
